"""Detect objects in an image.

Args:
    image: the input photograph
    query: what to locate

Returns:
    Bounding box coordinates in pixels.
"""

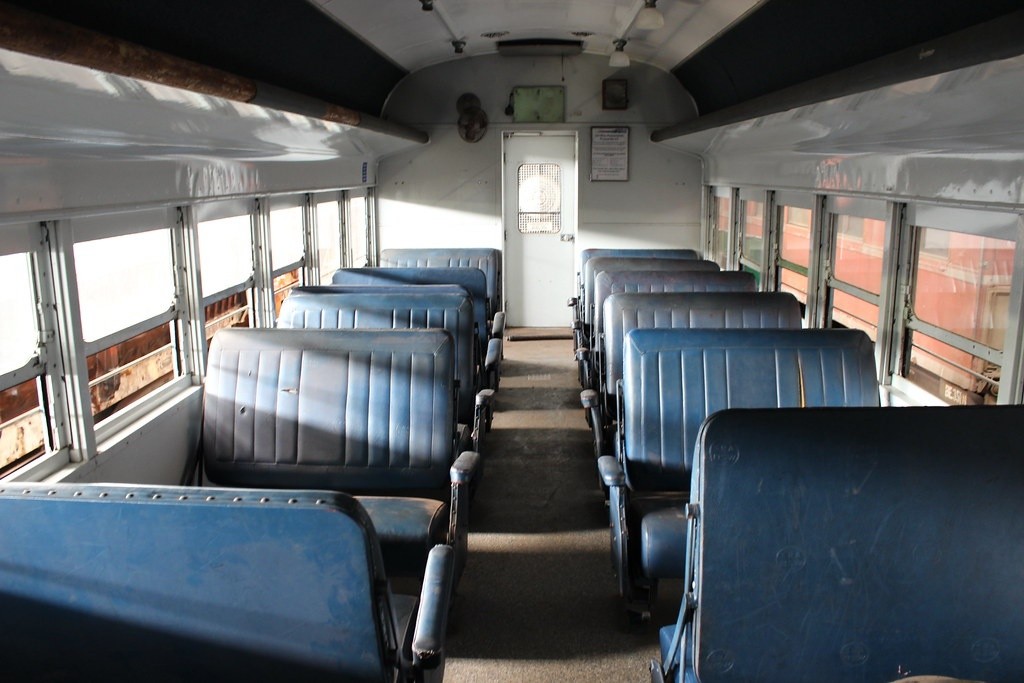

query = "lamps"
[608,39,630,68]
[635,0,664,29]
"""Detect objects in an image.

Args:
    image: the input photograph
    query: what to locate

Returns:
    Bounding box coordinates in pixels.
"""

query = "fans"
[456,92,488,143]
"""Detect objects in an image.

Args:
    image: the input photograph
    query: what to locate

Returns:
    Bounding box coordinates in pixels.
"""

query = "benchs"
[598,327,882,629]
[0,479,458,683]
[330,267,503,400]
[584,249,702,260]
[181,328,485,633]
[380,248,503,317]
[579,292,805,491]
[573,256,720,353]
[652,404,1024,683]
[582,272,759,431]
[278,283,497,502]
